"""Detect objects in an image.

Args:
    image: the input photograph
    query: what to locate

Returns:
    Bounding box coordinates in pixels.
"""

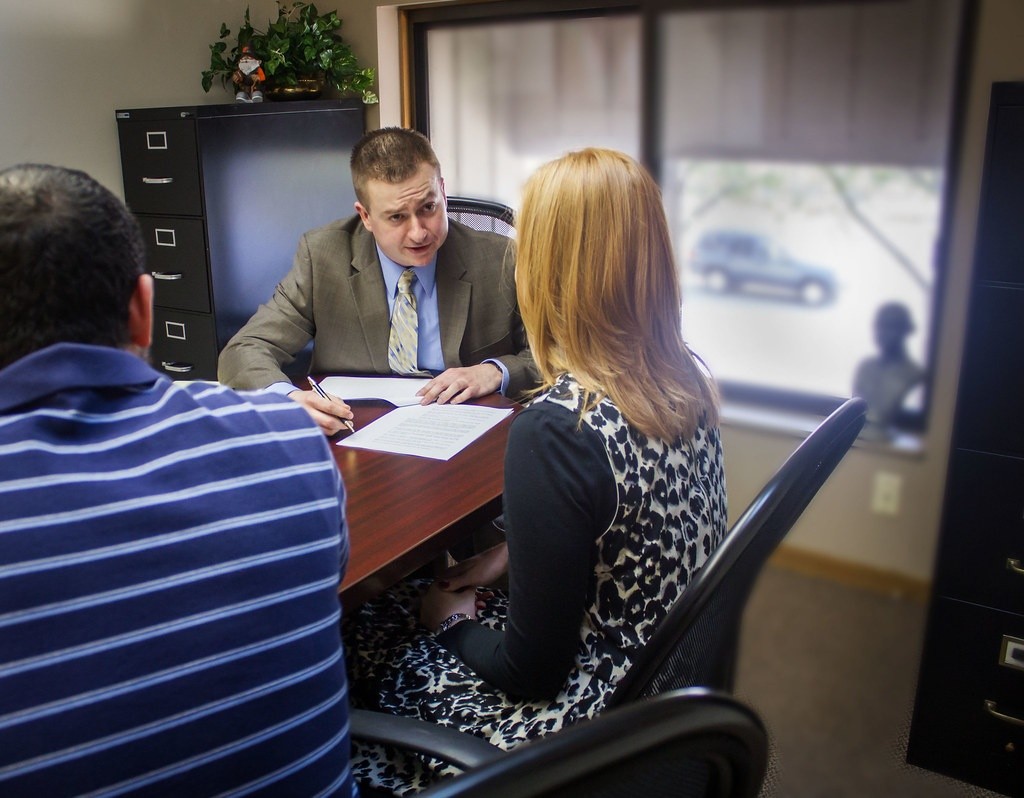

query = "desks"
[304,367,529,597]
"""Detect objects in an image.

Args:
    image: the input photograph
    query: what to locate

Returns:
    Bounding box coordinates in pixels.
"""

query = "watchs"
[435,612,475,637]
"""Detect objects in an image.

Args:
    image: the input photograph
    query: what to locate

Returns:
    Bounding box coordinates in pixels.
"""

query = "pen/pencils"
[307,376,355,434]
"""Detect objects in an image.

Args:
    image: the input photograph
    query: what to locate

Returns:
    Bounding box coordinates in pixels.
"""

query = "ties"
[389,269,434,377]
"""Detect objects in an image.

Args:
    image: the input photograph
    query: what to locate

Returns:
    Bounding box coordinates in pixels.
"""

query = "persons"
[340,146,727,798]
[845,300,928,440]
[0,161,363,798]
[216,124,544,605]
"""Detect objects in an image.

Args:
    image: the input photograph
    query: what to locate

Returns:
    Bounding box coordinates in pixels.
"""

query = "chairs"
[348,397,866,774]
[301,686,768,798]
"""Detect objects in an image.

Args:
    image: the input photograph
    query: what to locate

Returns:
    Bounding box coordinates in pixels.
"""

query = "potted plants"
[196,1,377,104]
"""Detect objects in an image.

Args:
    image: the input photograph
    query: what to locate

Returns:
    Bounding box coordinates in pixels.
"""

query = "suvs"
[689,228,840,306]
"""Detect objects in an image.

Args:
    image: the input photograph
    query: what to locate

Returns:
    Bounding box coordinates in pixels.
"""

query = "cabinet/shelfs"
[113,103,366,383]
[899,79,1024,796]
